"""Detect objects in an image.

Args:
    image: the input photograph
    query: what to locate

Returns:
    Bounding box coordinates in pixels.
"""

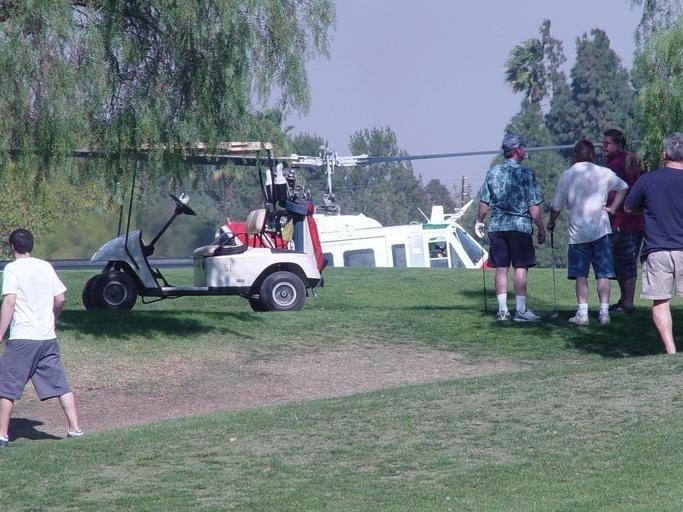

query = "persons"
[602,129,646,311]
[475,136,546,322]
[623,133,683,355]
[546,141,629,325]
[0,229,84,445]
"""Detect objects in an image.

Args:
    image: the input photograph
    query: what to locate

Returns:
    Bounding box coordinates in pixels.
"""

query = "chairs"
[191,208,267,257]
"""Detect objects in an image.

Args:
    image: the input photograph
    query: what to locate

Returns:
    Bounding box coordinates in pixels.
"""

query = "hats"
[502,134,529,151]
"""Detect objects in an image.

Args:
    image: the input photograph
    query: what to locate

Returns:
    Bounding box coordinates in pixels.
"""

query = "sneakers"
[569,312,589,325]
[0,434,8,448]
[496,309,511,320]
[614,305,635,312]
[63,430,83,439]
[598,315,610,325]
[513,309,541,321]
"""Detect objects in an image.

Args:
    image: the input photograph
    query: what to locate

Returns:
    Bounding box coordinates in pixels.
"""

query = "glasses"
[604,141,614,147]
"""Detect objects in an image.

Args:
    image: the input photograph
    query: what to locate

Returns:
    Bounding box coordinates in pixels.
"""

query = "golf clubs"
[480,228,489,311]
[551,231,559,318]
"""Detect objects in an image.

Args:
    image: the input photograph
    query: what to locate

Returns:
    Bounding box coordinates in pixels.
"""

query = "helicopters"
[2,136,645,270]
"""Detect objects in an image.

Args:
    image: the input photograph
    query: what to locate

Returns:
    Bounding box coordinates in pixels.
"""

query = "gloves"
[475,223,486,238]
